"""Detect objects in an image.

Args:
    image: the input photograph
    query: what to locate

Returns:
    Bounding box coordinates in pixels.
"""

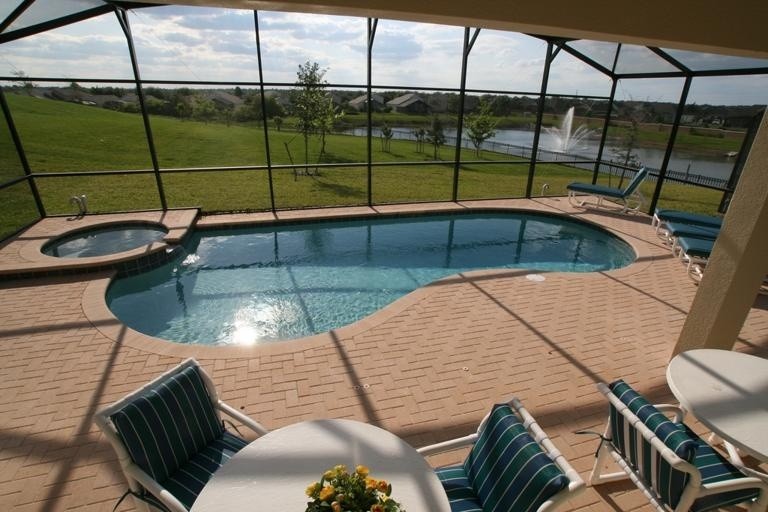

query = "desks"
[667,348,768,462]
[186,420,452,512]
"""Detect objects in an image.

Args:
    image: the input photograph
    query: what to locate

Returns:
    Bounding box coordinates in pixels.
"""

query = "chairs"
[95,357,271,512]
[566,165,649,215]
[590,379,768,512]
[652,207,768,294]
[416,396,587,512]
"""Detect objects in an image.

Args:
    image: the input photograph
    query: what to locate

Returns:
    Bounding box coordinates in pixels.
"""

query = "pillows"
[463,403,570,512]
[609,381,697,512]
[111,367,222,494]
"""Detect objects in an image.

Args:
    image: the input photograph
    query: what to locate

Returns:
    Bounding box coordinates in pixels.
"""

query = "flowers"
[304,463,405,512]
